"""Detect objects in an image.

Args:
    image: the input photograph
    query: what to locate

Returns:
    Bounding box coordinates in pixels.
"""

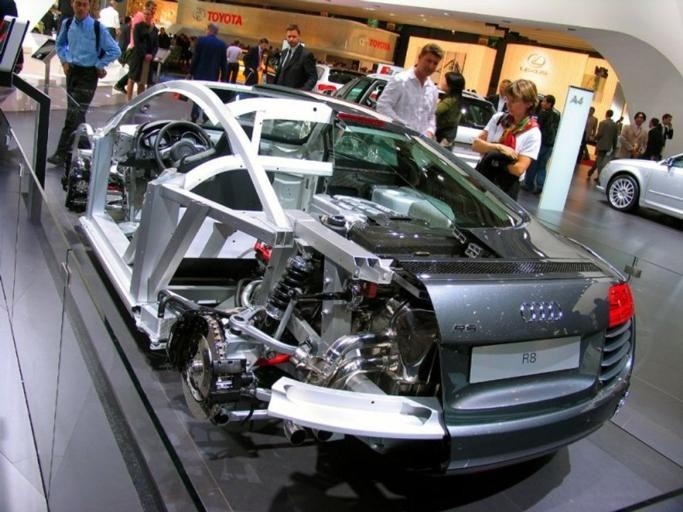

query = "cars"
[328,73,527,184]
[311,63,351,96]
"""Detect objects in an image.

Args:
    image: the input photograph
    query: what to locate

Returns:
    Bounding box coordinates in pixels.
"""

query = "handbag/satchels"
[127,48,134,64]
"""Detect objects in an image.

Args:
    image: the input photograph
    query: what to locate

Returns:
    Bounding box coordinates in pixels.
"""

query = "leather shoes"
[594,179,599,182]
[588,170,592,178]
[48,154,71,165]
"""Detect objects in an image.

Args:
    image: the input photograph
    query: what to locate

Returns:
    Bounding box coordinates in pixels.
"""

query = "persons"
[521,94,561,194]
[46,0,122,163]
[224,36,240,83]
[577,107,599,163]
[42,5,62,34]
[242,38,268,85]
[184,22,230,82]
[471,75,527,200]
[646,113,673,160]
[620,111,645,157]
[113,1,156,96]
[483,79,512,113]
[273,24,319,92]
[587,109,618,185]
[121,15,130,57]
[437,71,465,152]
[158,27,169,47]
[377,42,444,138]
[648,116,659,132]
[99,0,120,41]
[124,9,156,101]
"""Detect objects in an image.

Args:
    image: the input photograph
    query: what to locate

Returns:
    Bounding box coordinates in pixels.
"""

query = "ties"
[260,52,262,65]
[284,51,293,67]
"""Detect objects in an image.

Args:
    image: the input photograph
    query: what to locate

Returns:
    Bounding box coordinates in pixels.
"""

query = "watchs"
[494,143,503,153]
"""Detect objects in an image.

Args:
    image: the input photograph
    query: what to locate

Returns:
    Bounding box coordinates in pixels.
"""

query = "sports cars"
[76,78,637,474]
[596,152,682,222]
